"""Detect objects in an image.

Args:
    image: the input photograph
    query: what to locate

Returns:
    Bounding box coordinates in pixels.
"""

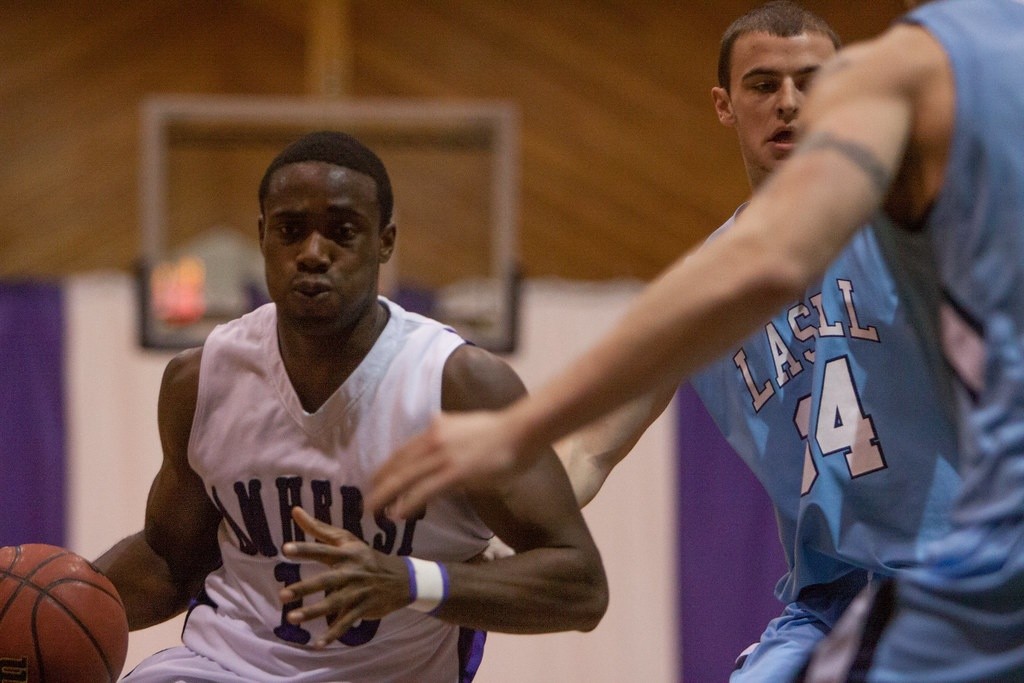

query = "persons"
[361,0,1024,683]
[93,127,609,683]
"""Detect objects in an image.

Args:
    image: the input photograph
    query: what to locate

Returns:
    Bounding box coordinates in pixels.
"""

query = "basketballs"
[0,542,131,683]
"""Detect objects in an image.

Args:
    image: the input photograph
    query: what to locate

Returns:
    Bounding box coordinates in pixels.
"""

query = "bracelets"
[406,553,446,616]
